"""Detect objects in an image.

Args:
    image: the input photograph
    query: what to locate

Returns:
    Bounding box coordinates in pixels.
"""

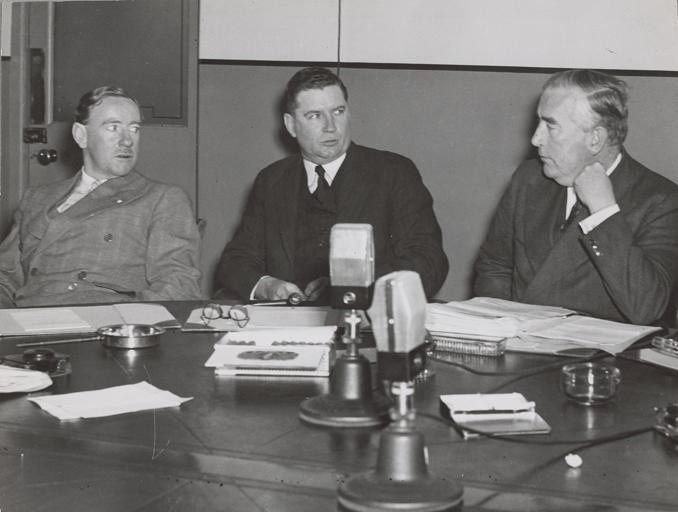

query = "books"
[439,392,555,440]
[421,299,665,359]
[637,337,678,372]
[204,324,339,376]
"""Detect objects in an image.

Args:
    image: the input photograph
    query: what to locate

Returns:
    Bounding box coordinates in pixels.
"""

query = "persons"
[1,84,205,310]
[212,65,449,309]
[470,68,677,348]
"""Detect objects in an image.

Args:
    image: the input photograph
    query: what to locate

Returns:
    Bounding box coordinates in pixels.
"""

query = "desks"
[0,299,677,512]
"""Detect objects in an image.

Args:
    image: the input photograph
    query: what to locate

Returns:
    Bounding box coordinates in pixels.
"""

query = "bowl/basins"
[560,362,622,406]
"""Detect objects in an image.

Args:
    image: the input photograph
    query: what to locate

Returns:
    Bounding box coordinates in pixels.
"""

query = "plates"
[203,324,338,370]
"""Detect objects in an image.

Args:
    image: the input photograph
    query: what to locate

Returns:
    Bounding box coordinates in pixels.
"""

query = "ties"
[302,163,339,282]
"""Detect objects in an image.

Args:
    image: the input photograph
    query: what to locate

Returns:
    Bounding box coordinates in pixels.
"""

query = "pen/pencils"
[454,409,527,414]
